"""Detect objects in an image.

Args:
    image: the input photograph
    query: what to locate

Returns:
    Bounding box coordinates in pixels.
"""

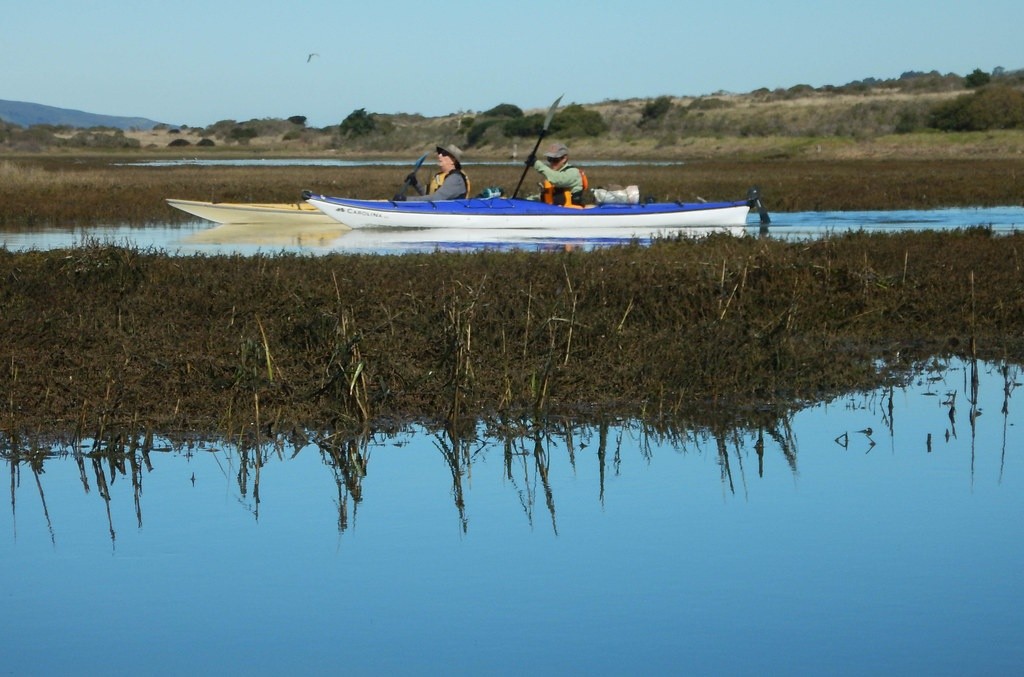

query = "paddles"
[510,91,566,199]
[390,151,429,201]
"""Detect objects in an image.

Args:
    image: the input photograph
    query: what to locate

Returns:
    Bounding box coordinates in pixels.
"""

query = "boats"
[334,229,742,247]
[166,199,341,225]
[163,224,352,249]
[303,188,758,231]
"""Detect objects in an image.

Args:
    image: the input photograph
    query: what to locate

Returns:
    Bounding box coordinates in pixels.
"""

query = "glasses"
[438,150,449,156]
[546,156,561,163]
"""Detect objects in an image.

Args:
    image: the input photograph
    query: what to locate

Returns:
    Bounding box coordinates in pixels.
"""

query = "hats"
[436,144,464,171]
[544,143,568,158]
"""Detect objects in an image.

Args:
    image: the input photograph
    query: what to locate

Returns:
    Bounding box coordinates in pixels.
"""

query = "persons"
[392,143,471,201]
[524,143,588,208]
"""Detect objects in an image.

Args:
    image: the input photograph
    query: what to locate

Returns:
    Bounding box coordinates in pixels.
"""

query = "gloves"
[524,154,537,167]
[393,194,407,201]
[404,172,418,186]
